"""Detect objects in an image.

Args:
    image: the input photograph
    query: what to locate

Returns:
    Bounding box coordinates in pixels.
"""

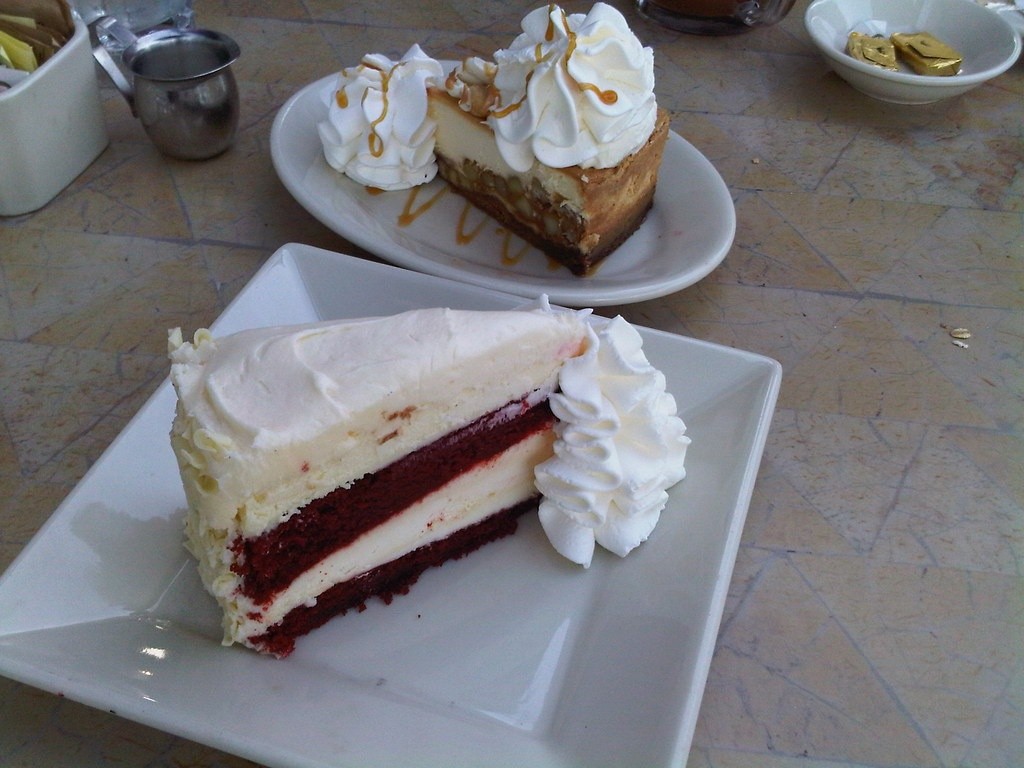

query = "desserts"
[166,294,693,658]
[315,2,671,276]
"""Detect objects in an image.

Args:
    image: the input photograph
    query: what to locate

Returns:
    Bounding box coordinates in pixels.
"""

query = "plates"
[0,243,782,768]
[269,61,736,304]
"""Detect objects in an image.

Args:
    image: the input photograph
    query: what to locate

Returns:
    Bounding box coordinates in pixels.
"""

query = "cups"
[87,5,239,160]
[0,10,109,215]
[637,0,796,36]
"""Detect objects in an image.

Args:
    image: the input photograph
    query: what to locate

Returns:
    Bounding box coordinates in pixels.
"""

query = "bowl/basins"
[805,0,1022,105]
